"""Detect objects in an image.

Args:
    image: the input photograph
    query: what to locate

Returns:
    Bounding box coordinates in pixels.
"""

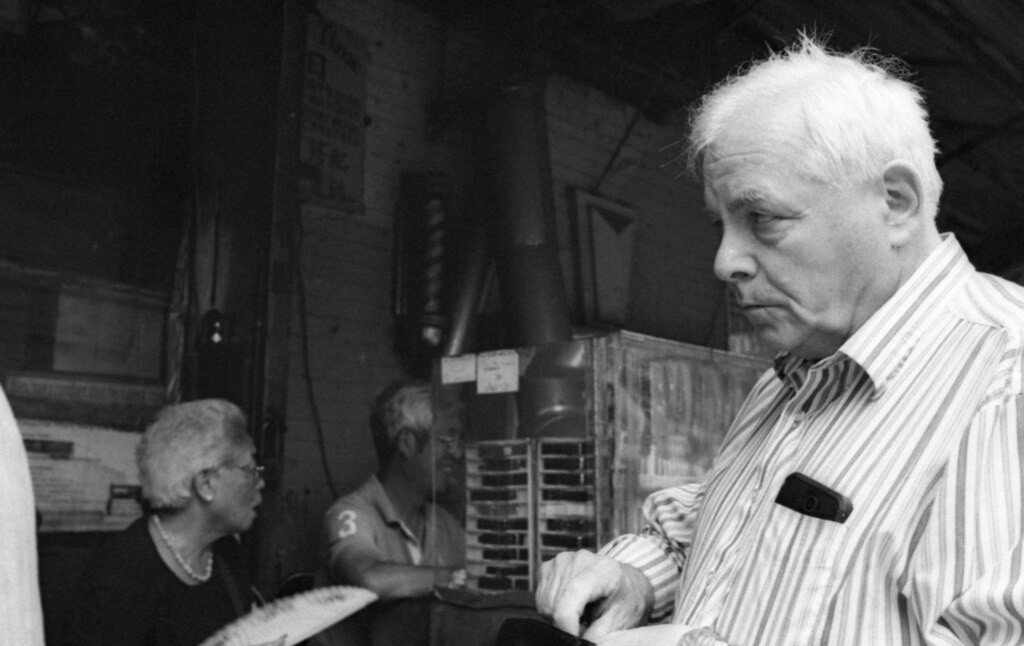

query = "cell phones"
[775,472,853,524]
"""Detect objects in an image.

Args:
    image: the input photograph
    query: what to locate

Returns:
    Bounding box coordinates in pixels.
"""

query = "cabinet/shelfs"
[1,261,184,537]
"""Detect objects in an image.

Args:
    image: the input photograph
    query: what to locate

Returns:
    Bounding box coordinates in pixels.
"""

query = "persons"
[0,377,51,645]
[535,28,1023,646]
[78,397,298,646]
[316,373,472,646]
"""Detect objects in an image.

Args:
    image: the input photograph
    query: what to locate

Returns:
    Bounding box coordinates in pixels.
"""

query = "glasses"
[422,433,472,453]
[226,462,266,482]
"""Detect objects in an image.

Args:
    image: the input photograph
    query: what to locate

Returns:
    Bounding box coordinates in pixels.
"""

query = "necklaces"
[153,509,219,585]
